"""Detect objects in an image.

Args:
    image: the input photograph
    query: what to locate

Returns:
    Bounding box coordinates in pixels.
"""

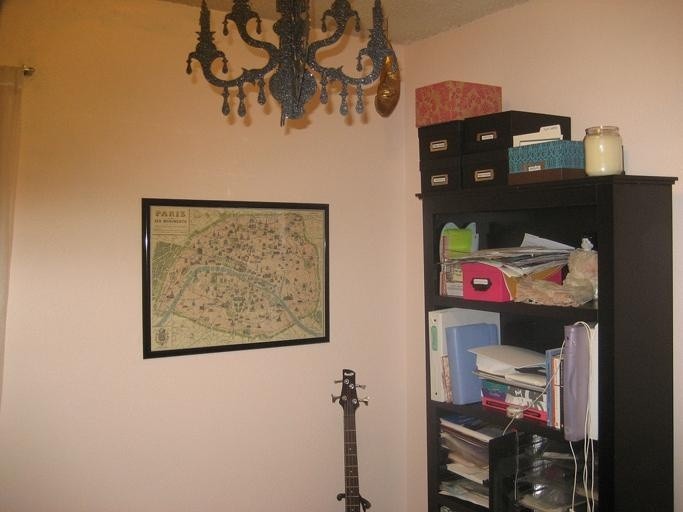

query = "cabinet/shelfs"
[419,174,679,511]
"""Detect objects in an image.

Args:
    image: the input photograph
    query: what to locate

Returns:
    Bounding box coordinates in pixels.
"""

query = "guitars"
[332,370,371,512]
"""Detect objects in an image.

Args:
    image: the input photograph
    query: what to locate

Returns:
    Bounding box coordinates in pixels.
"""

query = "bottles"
[584,126,623,178]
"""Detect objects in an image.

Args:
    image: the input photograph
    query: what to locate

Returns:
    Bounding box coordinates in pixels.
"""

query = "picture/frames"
[140,197,332,360]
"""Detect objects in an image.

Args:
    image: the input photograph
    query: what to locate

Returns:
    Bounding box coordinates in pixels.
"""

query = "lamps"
[184,0,404,131]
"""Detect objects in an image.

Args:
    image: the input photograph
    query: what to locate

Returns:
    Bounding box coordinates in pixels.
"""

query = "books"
[544,319,599,443]
[436,411,512,508]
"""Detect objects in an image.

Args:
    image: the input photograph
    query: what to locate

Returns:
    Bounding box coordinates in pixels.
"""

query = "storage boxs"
[416,81,587,193]
